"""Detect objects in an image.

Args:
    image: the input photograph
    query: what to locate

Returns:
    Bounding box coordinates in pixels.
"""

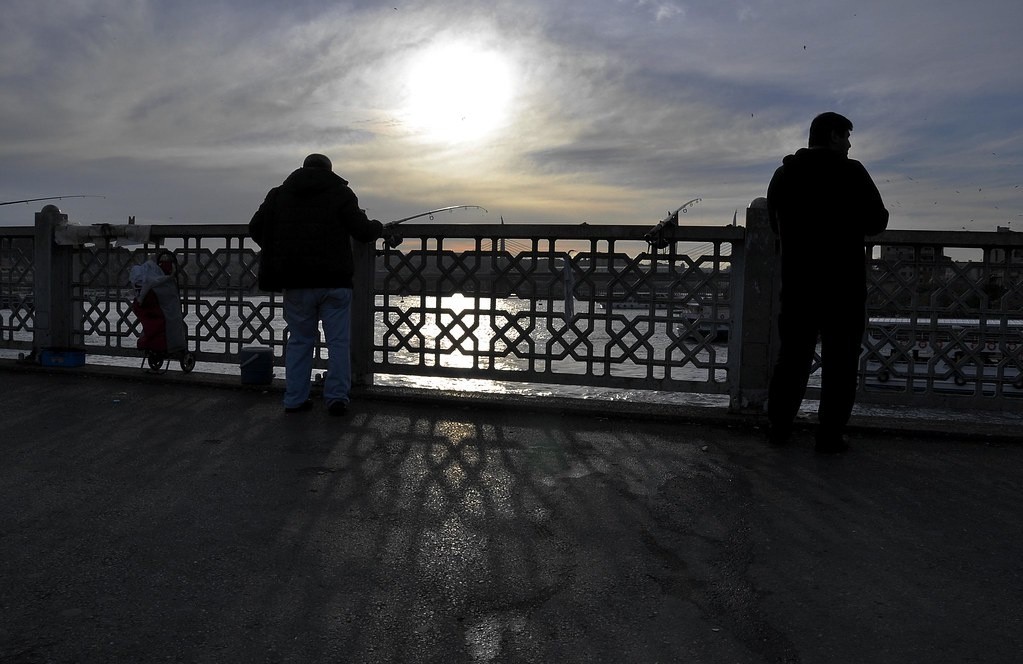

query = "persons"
[249,154,384,416]
[766,112,890,455]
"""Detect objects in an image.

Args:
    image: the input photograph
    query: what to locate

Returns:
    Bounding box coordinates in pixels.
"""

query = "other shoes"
[326,397,351,416]
[286,398,314,414]
[814,434,850,458]
[764,424,788,440]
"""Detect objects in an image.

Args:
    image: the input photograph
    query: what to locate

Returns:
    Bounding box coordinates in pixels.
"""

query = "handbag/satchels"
[258,188,283,292]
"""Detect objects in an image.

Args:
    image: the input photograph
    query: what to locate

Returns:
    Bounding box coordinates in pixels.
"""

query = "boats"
[593,288,724,310]
[675,297,729,343]
[857,317,1023,397]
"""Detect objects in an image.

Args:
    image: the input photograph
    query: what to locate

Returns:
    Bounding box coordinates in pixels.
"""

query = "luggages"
[128,250,196,375]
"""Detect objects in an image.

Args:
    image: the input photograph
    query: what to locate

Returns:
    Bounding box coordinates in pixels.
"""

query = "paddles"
[383,205,489,229]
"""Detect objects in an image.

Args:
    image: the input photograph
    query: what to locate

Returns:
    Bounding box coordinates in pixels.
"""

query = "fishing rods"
[644,195,702,242]
[0,194,105,206]
